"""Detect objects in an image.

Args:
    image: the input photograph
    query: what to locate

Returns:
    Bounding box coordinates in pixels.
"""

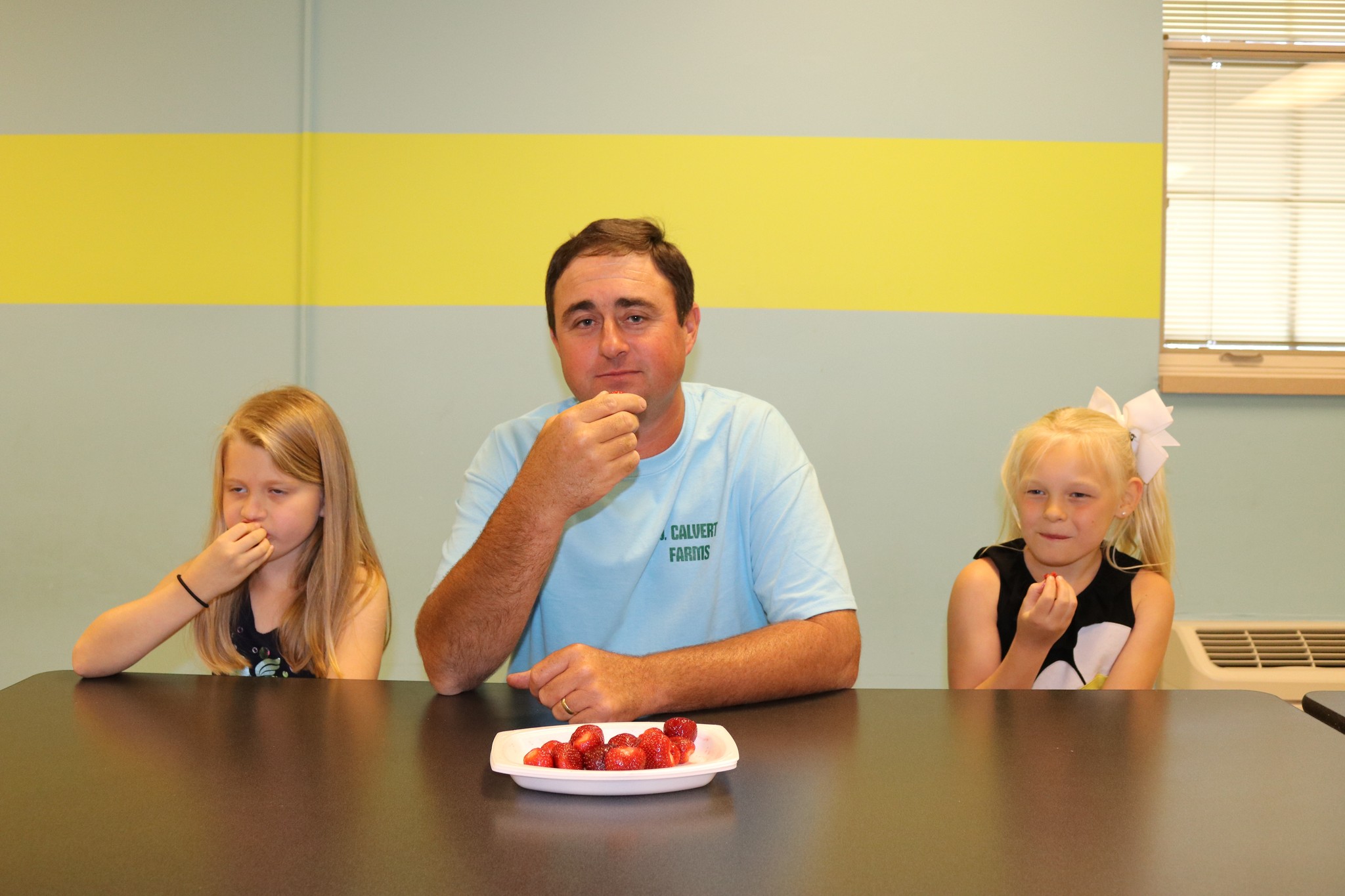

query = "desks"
[0,669,1345,896]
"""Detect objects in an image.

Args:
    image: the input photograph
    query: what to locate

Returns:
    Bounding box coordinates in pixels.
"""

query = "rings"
[561,697,579,715]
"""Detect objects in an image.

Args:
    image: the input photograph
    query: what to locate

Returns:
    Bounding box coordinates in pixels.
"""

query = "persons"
[414,217,861,725]
[946,386,1181,689]
[72,386,393,680]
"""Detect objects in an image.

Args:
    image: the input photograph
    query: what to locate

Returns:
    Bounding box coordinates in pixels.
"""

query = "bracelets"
[177,574,209,608]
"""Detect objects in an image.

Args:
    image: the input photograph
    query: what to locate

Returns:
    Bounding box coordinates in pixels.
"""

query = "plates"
[489,721,739,796]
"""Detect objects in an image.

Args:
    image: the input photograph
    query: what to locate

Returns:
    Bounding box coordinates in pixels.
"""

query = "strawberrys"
[1044,572,1057,579]
[523,718,697,770]
[608,391,625,394]
